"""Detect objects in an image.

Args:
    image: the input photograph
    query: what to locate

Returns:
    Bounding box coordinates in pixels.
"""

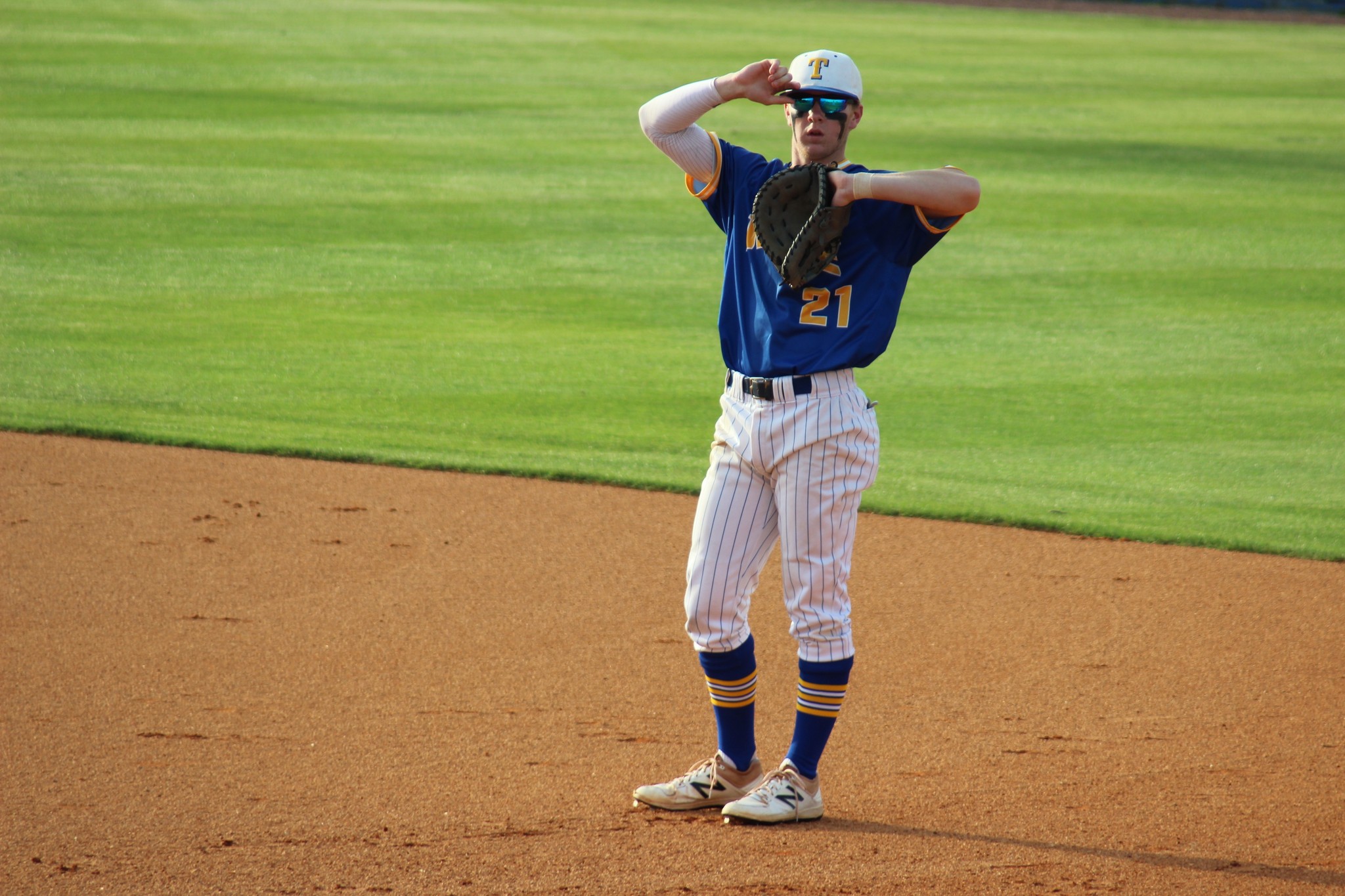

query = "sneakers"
[722,768,824,824]
[634,749,762,809]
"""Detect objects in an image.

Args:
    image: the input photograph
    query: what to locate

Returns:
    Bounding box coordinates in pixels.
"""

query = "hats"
[779,50,862,105]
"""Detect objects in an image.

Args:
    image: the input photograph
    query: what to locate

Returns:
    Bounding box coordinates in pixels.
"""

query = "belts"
[727,369,811,400]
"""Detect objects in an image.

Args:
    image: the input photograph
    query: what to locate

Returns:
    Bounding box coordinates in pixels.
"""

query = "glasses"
[786,93,855,114]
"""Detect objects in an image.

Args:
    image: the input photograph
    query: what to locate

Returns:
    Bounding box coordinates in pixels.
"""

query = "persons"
[634,48,980,824]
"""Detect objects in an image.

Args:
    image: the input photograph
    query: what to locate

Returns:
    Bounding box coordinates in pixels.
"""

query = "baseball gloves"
[751,162,852,289]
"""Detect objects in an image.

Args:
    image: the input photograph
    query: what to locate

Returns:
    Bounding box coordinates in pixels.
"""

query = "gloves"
[867,398,879,409]
